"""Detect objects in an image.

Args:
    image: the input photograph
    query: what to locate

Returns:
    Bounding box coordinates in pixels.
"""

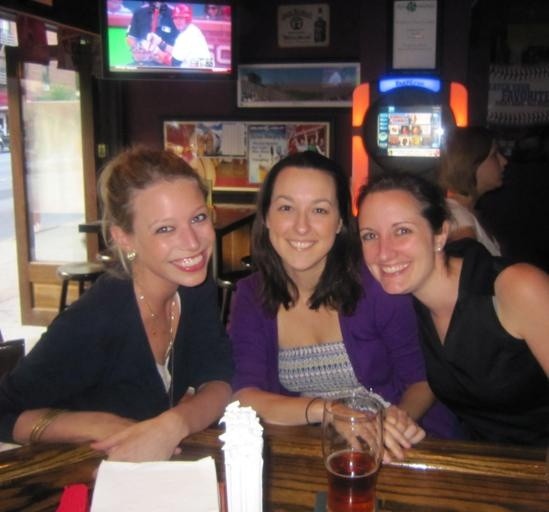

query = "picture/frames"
[388,0,444,74]
[236,62,362,108]
[159,113,338,205]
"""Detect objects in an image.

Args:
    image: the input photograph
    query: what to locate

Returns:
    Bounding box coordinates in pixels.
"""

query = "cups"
[326,493,378,512]
[322,392,386,491]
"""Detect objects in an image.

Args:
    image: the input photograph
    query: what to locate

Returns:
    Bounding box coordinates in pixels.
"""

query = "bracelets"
[29,407,68,444]
[305,397,321,425]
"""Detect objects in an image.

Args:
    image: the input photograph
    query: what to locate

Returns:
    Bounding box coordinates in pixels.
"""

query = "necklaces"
[139,292,176,408]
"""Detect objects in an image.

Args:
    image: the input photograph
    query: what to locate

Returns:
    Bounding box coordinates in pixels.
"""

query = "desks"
[79,208,257,311]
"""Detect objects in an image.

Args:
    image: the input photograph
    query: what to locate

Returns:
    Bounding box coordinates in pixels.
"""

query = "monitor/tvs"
[101,0,237,82]
[375,104,444,157]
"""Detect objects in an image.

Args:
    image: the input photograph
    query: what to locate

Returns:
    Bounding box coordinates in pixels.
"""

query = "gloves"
[143,32,165,51]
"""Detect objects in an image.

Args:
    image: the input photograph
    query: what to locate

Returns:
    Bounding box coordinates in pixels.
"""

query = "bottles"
[388,115,422,148]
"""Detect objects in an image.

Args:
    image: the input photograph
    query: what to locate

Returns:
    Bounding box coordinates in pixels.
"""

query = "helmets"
[172,3,192,23]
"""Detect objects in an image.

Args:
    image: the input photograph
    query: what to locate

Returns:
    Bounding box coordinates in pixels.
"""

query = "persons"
[106,0,231,69]
[0,143,234,480]
[294,129,327,156]
[227,151,460,464]
[437,128,508,257]
[357,170,549,447]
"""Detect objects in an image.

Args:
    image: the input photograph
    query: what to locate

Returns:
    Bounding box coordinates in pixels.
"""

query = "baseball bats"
[151,8,159,32]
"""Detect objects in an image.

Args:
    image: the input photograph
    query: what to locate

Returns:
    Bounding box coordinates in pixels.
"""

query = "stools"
[95,250,118,264]
[240,256,255,269]
[57,263,105,313]
[216,269,254,326]
